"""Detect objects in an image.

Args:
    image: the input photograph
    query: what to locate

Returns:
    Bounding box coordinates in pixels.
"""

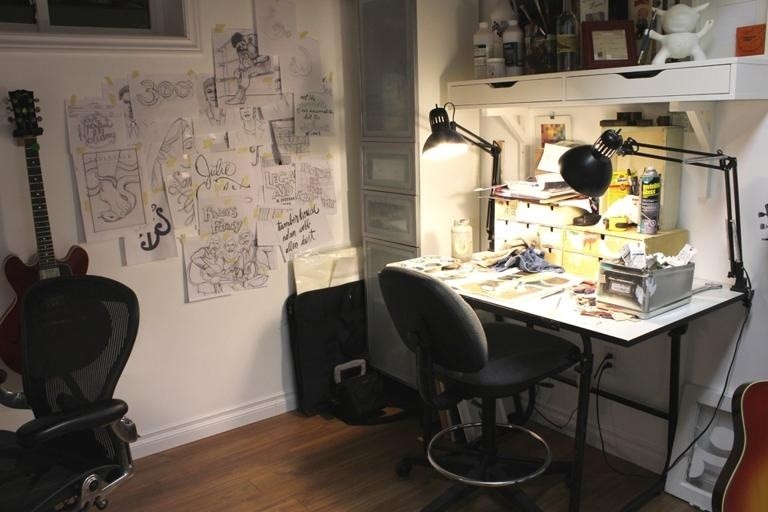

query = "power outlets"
[600,344,620,374]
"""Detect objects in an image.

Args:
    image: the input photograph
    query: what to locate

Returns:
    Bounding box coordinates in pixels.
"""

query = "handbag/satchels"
[335,371,384,424]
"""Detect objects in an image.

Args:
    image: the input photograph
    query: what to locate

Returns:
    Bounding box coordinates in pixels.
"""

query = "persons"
[216,236,244,290]
[190,235,225,293]
[225,32,273,106]
[238,232,270,290]
[235,103,268,146]
[201,78,229,129]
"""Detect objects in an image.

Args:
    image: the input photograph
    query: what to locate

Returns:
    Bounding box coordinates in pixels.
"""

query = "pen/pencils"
[514,282,523,290]
[627,169,636,195]
[638,10,657,64]
[541,290,564,299]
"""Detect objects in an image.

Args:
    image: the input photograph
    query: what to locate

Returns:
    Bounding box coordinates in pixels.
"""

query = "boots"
[225,87,246,104]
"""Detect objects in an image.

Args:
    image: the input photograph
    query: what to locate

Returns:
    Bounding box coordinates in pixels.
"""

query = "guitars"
[2,90,113,377]
[712,201,768,511]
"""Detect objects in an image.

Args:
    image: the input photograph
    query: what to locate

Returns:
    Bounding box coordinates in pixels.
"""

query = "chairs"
[376,265,581,512]
[0,276,143,512]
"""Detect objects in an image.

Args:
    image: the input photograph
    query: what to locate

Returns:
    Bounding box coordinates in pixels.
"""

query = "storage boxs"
[597,262,695,320]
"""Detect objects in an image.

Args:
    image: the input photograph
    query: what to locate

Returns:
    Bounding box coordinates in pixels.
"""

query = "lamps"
[557,128,751,294]
[421,101,503,251]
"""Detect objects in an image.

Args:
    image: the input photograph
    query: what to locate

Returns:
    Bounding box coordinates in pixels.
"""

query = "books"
[492,172,581,205]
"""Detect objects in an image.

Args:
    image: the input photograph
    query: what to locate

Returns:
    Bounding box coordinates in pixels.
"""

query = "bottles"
[556,0,581,75]
[451,216,474,263]
[472,19,525,79]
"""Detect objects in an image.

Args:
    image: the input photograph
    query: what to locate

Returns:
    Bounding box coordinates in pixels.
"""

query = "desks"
[383,257,753,512]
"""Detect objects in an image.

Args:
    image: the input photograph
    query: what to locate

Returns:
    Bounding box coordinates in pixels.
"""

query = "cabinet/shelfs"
[353,2,479,389]
[444,55,768,108]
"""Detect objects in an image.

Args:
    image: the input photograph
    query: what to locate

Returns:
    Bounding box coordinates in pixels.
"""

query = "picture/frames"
[581,20,639,70]
